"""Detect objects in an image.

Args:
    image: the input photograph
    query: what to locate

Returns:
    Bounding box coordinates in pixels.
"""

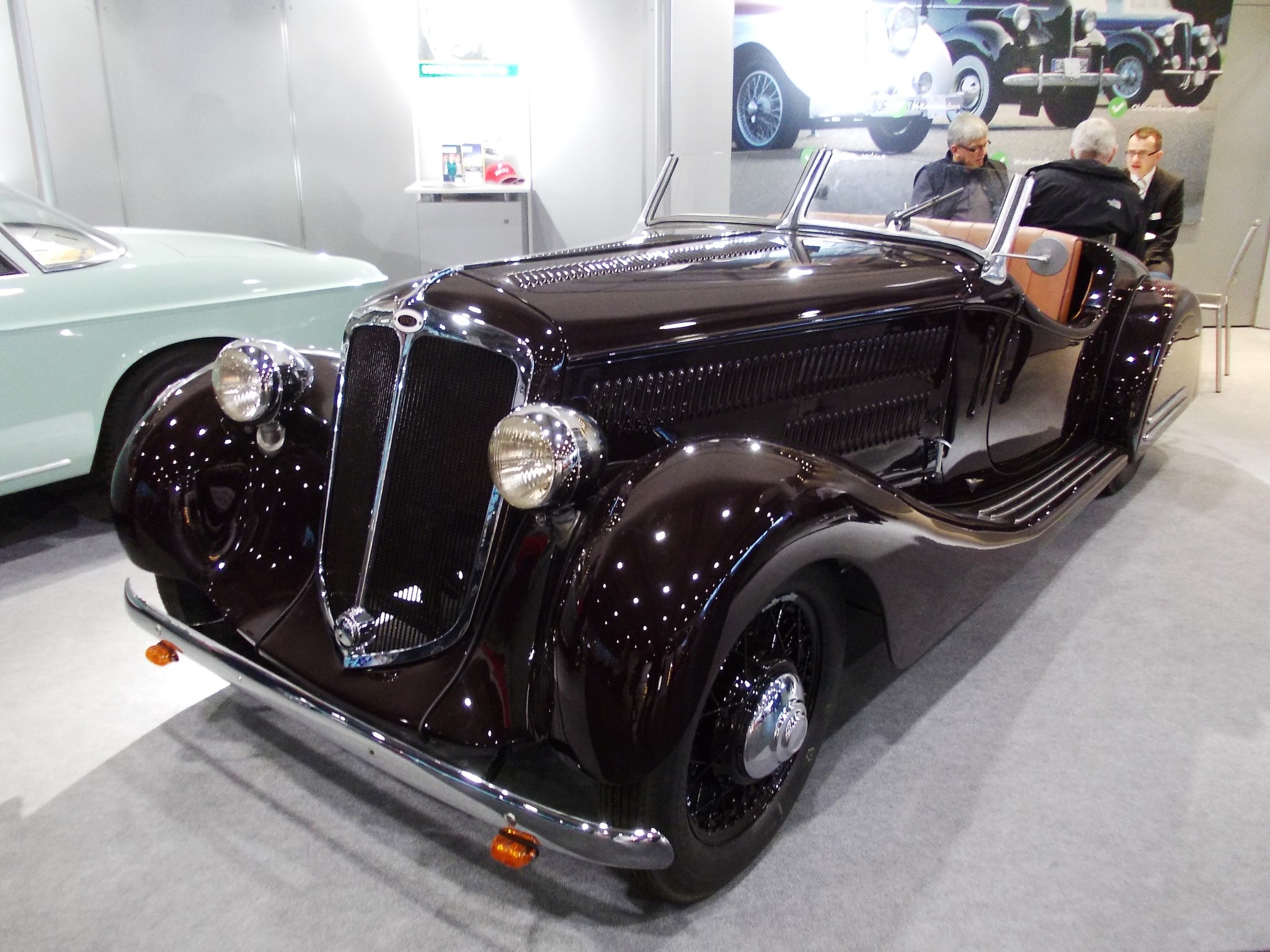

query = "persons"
[1017,117,1148,265]
[1117,127,1184,280]
[905,114,1010,224]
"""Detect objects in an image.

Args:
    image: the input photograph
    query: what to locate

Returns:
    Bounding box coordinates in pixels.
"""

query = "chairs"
[1193,219,1264,395]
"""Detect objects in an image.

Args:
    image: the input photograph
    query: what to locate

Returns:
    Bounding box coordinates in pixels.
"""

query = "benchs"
[766,211,1083,326]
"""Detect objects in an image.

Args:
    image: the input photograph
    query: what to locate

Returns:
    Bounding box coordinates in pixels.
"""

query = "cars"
[0,181,390,497]
[735,0,1224,156]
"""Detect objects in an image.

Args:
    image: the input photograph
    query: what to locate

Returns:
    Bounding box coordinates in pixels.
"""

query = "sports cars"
[105,147,1205,904]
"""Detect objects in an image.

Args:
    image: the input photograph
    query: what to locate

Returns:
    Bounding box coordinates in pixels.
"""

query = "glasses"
[1123,149,1160,159]
[956,140,992,154]
